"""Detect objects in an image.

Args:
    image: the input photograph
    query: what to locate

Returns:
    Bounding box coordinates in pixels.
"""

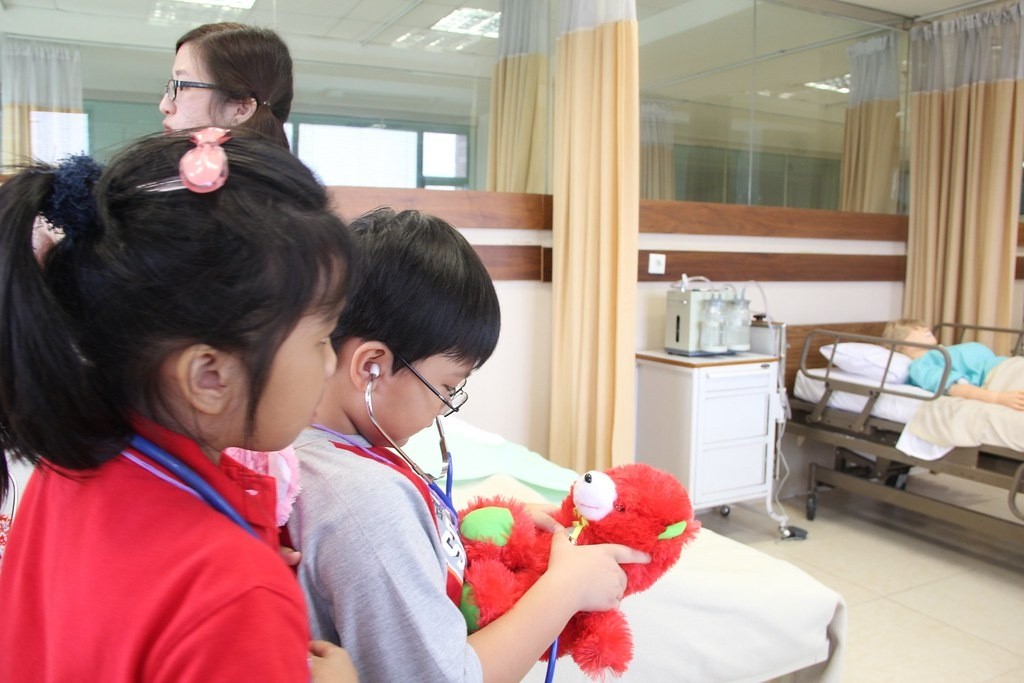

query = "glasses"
[165,79,261,106]
[370,336,468,418]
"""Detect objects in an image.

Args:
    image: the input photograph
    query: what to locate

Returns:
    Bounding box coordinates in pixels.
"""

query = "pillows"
[819,342,914,387]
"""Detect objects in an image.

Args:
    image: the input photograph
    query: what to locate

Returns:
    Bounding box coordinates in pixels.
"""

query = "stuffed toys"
[458,464,703,678]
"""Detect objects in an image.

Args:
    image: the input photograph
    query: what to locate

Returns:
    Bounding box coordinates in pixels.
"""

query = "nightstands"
[633,351,781,517]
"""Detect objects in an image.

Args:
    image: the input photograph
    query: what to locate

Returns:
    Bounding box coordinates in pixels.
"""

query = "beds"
[779,317,1023,546]
[386,415,848,682]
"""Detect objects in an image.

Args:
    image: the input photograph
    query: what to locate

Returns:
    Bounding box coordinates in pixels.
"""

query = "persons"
[882,319,1024,411]
[0,126,357,683]
[279,208,652,682]
[158,22,293,152]
